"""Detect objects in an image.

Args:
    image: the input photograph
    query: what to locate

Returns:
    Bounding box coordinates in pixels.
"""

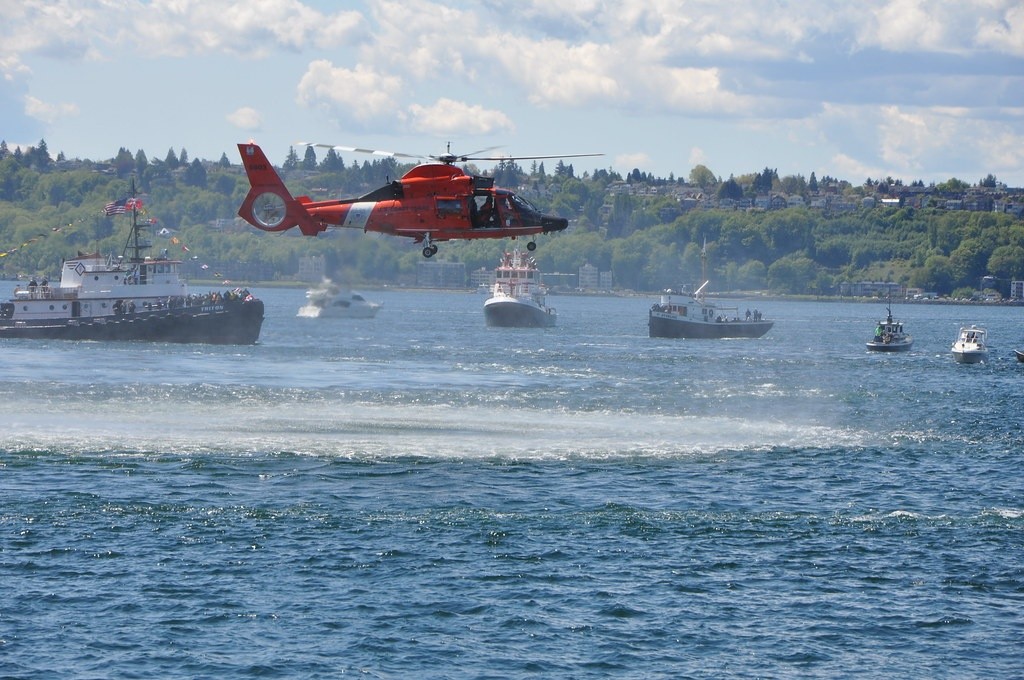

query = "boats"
[483,236,558,328]
[648,235,774,339]
[1015,349,1024,362]
[296,279,382,319]
[950,324,989,363]
[0,178,266,345]
[866,284,915,352]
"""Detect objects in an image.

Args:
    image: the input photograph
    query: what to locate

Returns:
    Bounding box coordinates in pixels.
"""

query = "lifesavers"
[166,315,174,321]
[120,319,128,326]
[93,322,100,329]
[107,321,113,328]
[709,310,713,315]
[134,317,142,326]
[79,322,86,328]
[148,316,158,323]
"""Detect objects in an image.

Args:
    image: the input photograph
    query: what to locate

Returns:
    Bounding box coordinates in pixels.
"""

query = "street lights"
[312,256,316,272]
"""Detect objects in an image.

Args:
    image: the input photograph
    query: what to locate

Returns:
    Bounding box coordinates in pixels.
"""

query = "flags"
[0,198,244,296]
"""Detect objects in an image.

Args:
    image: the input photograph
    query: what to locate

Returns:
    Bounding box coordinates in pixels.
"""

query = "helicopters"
[237,141,606,258]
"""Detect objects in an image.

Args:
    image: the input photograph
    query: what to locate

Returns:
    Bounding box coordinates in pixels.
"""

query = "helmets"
[486,196,493,201]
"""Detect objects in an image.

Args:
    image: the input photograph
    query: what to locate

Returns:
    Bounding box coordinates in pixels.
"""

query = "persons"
[157,287,250,310]
[745,308,761,322]
[474,196,494,227]
[28,276,49,292]
[875,325,891,343]
[120,300,136,315]
